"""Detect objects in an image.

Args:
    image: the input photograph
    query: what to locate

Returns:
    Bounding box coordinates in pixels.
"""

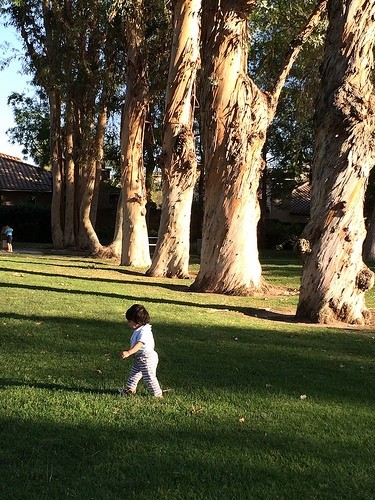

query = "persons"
[3,225,14,253]
[119,304,164,399]
